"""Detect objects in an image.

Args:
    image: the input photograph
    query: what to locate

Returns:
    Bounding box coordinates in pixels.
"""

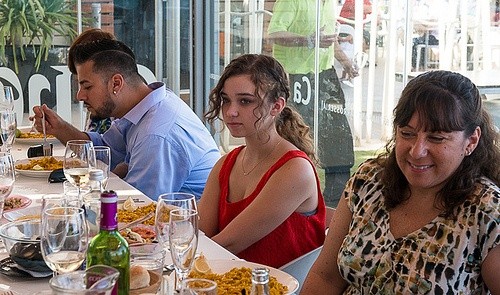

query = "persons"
[33,50,221,210]
[298,71,500,295]
[267,0,360,210]
[188,55,326,269]
[337,0,372,81]
[67,29,158,133]
[409,23,439,68]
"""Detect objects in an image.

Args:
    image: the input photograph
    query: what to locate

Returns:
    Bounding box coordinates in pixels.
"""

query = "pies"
[118,202,175,225]
[20,133,55,138]
[187,267,289,295]
[15,155,64,169]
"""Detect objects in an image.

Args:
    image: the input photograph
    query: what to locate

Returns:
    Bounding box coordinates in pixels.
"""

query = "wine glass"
[169,210,198,294]
[155,192,198,294]
[0,86,112,214]
[341,51,368,87]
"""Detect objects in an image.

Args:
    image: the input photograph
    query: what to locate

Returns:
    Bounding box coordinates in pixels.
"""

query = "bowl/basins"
[0,219,51,271]
[130,270,162,294]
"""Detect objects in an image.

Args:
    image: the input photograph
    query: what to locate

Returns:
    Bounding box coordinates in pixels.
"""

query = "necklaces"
[242,138,282,176]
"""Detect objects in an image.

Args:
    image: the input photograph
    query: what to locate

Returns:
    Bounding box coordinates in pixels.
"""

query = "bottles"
[85,190,131,295]
[82,170,106,270]
[248,268,272,295]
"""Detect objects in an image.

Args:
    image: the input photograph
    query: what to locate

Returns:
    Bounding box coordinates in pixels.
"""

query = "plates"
[11,126,60,143]
[2,195,33,214]
[190,259,299,294]
[117,203,189,226]
[3,206,42,223]
[14,157,87,178]
[116,225,156,256]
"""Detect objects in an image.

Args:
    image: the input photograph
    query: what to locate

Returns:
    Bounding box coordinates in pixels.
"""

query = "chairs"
[278,242,323,295]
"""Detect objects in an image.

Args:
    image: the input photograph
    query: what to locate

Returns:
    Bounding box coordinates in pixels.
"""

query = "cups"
[179,278,218,295]
[40,207,90,275]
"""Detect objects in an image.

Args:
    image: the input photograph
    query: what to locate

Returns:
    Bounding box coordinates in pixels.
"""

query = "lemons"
[16,129,21,137]
[123,197,135,210]
[33,165,43,170]
[194,252,213,272]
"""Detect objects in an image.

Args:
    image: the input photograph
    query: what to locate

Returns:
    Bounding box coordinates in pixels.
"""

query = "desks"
[0,124,239,295]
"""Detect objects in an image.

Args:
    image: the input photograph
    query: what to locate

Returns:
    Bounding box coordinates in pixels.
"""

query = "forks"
[40,111,52,157]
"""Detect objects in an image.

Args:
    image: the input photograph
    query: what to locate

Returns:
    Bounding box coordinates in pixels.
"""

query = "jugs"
[50,263,120,295]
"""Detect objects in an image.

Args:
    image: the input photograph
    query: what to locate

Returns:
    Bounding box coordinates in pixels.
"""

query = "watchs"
[307,36,314,49]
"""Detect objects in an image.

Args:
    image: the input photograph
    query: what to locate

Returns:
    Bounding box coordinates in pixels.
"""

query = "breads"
[130,266,150,289]
